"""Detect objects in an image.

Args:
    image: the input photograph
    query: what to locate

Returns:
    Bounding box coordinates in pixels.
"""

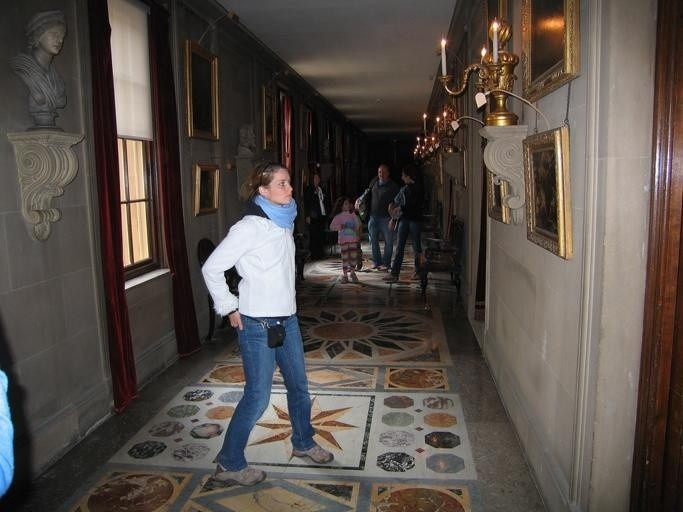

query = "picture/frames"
[520,124,576,262]
[483,166,511,225]
[258,86,278,154]
[189,161,221,218]
[297,104,310,152]
[183,46,220,142]
[300,167,309,196]
[519,0,581,107]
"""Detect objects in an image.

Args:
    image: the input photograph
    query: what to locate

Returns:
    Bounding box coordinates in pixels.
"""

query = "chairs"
[413,217,466,307]
[196,237,241,342]
[416,201,443,247]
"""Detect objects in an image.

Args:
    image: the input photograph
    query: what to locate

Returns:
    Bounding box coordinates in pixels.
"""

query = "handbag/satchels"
[353,187,373,225]
[266,322,287,351]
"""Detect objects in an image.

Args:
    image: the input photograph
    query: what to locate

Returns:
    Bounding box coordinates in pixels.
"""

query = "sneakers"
[410,271,421,280]
[340,275,348,284]
[350,272,359,284]
[292,443,335,465]
[212,465,268,488]
[382,273,400,283]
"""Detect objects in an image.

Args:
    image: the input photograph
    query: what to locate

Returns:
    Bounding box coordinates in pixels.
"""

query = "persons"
[330,196,364,282]
[17,7,71,130]
[354,165,400,271]
[350,201,366,271]
[382,168,423,283]
[237,124,255,156]
[0,369,17,498]
[302,172,330,262]
[200,161,335,486]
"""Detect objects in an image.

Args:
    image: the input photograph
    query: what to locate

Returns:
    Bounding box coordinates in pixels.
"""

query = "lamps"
[411,18,519,168]
